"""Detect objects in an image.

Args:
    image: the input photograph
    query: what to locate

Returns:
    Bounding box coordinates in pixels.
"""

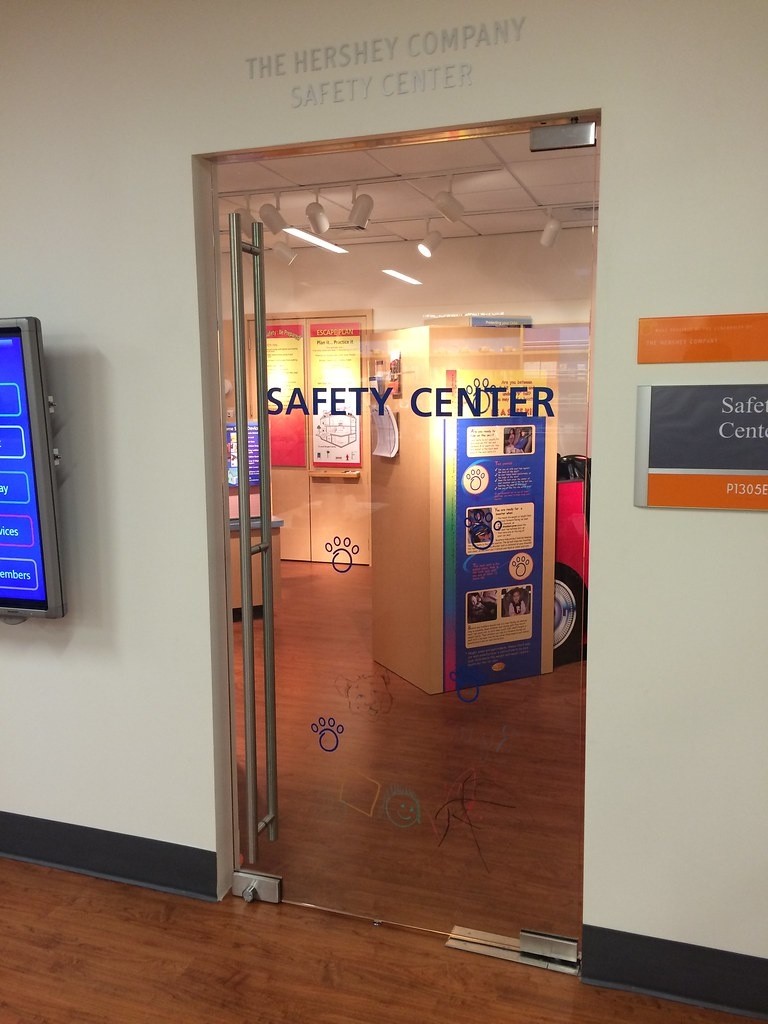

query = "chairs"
[513,428,532,453]
[503,588,529,616]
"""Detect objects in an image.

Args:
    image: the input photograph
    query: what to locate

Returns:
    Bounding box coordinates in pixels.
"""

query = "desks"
[230,516,286,609]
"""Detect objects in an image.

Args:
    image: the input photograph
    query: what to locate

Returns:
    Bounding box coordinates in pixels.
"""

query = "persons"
[509,587,526,616]
[471,593,486,615]
[469,511,491,543]
[504,428,529,454]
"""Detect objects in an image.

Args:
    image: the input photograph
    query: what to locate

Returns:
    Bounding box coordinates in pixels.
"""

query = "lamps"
[418,220,444,259]
[432,172,465,222]
[231,195,261,241]
[346,181,374,230]
[273,232,297,267]
[537,207,560,248]
[259,191,291,235]
[302,186,332,236]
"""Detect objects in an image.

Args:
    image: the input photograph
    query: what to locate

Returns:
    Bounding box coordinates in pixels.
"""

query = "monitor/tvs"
[0,316,67,620]
